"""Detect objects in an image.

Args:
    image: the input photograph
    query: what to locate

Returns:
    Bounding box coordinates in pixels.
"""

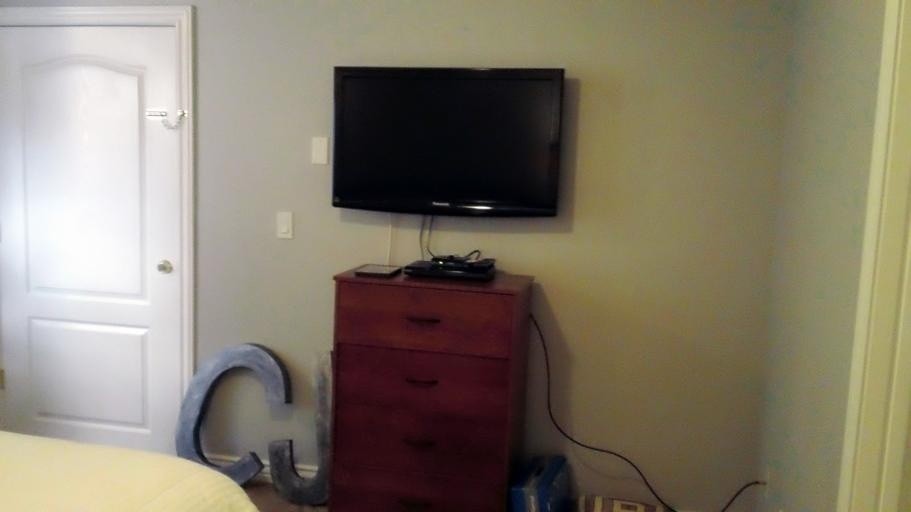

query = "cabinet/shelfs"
[327,262,536,512]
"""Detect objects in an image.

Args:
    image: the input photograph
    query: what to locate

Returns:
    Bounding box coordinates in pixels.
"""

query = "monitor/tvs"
[331,64,566,219]
